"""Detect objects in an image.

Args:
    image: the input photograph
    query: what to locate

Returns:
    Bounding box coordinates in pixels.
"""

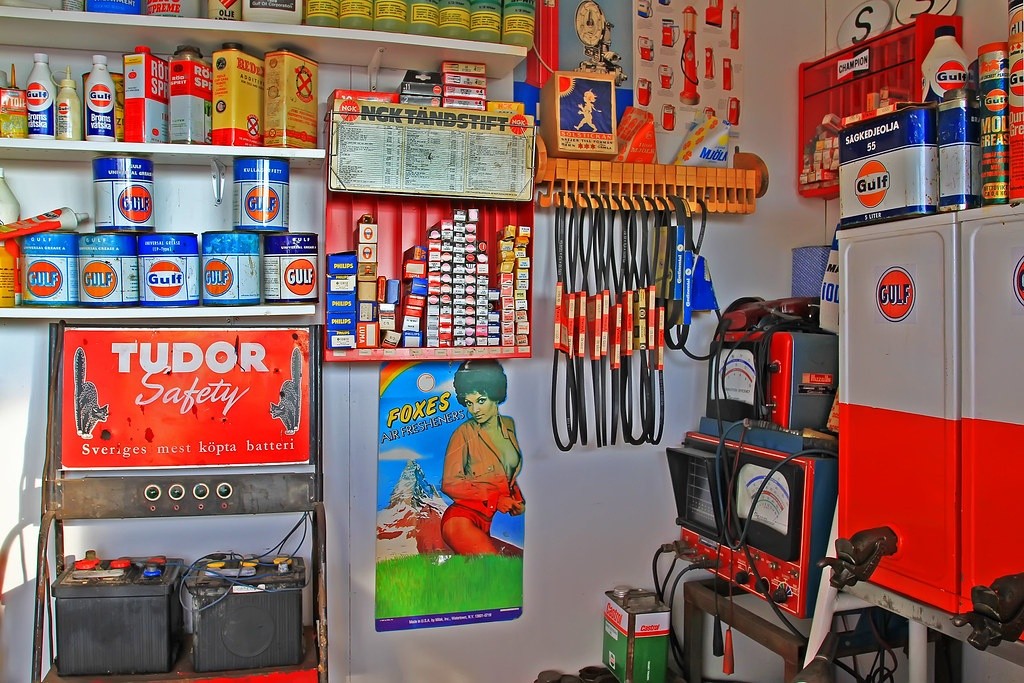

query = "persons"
[439,358,525,557]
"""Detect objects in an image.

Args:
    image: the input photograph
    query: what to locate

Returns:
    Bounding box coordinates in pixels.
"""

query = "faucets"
[816,526,898,590]
[953,572,1024,651]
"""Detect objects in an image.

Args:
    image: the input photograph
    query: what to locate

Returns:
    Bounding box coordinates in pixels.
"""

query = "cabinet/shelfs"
[0,0,535,320]
[798,14,963,200]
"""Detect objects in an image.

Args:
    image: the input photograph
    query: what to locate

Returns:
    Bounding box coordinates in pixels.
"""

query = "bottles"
[920,26,970,103]
[937,87,985,212]
[84,54,116,142]
[50,65,83,141]
[26,52,57,140]
[1,167,21,307]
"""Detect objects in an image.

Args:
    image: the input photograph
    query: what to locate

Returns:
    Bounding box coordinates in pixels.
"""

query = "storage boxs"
[539,71,619,161]
[837,106,940,227]
